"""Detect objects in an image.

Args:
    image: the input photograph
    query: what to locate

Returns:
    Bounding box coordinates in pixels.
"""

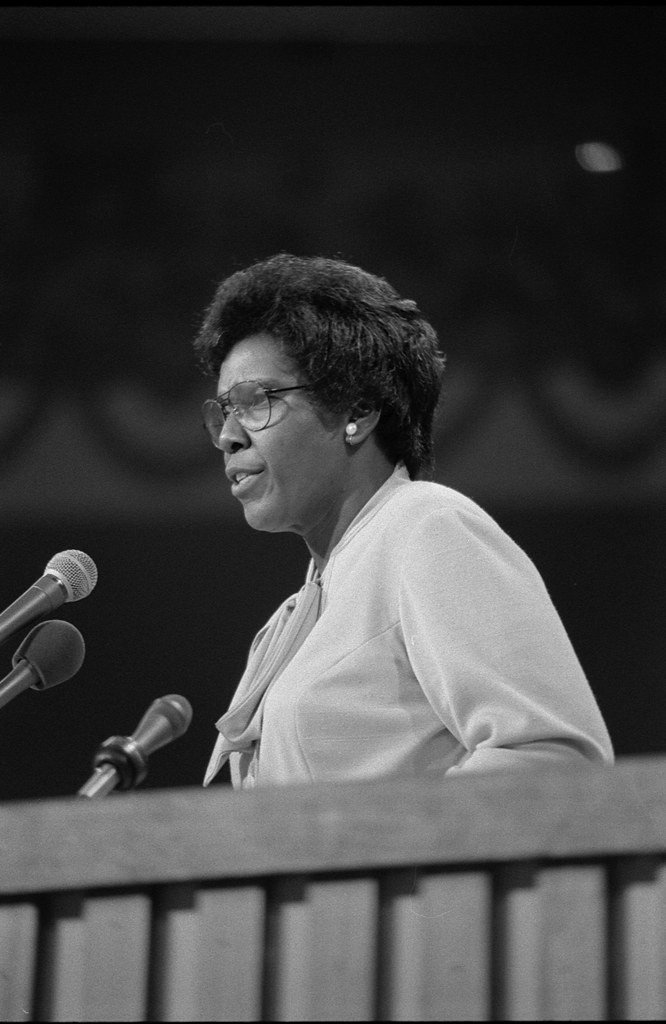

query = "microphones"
[0,550,98,644]
[0,620,86,710]
[78,693,193,801]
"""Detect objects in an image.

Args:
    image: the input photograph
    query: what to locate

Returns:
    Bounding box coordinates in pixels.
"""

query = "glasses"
[201,380,307,449]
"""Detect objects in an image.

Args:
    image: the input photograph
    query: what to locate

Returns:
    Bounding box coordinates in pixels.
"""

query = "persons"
[185,250,615,788]
